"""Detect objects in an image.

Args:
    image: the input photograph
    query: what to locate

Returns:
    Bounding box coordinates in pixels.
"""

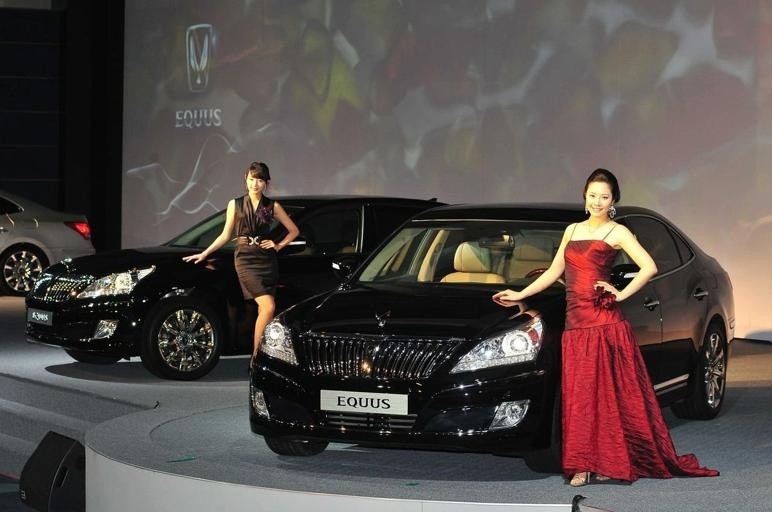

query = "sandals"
[570,472,590,486]
[594,474,611,482]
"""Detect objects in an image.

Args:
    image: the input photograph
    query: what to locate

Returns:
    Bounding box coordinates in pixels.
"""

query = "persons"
[181,160,300,371]
[489,167,721,487]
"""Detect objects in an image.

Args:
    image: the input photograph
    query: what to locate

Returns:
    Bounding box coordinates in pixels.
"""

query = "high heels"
[249,356,252,371]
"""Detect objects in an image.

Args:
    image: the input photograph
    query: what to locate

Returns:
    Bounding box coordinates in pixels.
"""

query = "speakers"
[18,431,84,512]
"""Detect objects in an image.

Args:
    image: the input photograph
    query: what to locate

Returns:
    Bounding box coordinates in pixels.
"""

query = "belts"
[236,237,266,245]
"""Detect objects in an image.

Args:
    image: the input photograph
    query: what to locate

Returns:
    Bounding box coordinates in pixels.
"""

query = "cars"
[0,194,96,297]
[25,197,447,380]
[249,204,734,474]
